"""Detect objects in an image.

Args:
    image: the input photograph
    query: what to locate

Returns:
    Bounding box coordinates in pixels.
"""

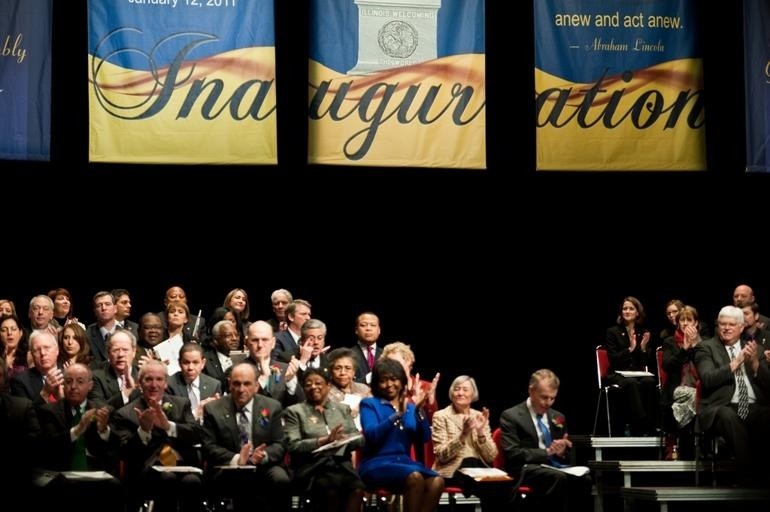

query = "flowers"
[551,414,565,429]
[164,402,174,415]
[257,407,271,427]
[271,363,285,382]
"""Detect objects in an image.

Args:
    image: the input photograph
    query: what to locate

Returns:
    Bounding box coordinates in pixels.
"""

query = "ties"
[238,405,251,448]
[119,373,126,390]
[104,332,112,343]
[308,357,316,369]
[729,345,750,421]
[366,346,375,370]
[536,412,572,469]
[70,405,89,471]
[187,382,199,412]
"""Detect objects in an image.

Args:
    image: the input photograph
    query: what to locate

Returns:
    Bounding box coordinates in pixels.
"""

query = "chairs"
[423,439,470,509]
[493,428,533,492]
[695,379,719,486]
[351,432,417,508]
[593,345,648,437]
[656,346,675,390]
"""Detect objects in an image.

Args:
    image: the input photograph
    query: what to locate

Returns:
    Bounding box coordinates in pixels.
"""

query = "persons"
[0,284,595,512]
[605,284,770,462]
